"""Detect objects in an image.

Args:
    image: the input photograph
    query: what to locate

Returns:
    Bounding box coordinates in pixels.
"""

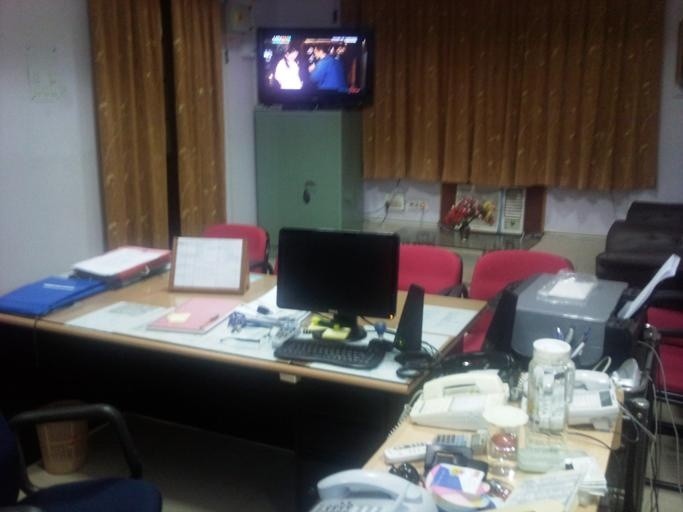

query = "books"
[146,298,241,334]
[72,245,169,288]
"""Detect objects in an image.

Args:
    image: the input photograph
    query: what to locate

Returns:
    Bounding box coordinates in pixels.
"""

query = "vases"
[460,222,470,242]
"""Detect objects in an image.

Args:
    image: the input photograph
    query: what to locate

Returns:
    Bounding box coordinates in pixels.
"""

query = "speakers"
[392,283,432,366]
[480,285,519,355]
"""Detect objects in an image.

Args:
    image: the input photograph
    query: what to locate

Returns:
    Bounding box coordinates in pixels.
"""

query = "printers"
[510,273,650,374]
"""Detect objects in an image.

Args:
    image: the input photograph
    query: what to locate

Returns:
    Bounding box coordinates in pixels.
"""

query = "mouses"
[397,365,423,378]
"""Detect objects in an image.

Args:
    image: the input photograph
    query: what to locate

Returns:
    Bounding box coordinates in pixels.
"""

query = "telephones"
[408,368,500,431]
[307,469,437,511]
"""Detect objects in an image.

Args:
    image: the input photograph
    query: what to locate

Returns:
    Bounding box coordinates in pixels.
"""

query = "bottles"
[524,338,573,472]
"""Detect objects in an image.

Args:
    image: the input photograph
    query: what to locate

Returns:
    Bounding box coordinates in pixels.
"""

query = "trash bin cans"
[34,399,89,476]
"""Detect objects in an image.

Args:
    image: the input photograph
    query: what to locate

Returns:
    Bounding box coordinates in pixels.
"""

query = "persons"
[308,45,350,93]
[275,47,303,90]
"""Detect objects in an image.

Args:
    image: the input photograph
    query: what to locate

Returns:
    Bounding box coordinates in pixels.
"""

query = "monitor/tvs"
[257,26,373,107]
[274,228,399,342]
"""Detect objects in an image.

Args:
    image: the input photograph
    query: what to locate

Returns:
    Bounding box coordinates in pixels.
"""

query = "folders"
[0,278,111,317]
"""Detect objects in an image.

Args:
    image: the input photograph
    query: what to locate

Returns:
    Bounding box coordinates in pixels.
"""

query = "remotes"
[433,432,468,454]
[383,441,427,465]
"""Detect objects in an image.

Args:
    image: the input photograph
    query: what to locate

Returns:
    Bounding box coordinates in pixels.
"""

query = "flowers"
[437,194,497,231]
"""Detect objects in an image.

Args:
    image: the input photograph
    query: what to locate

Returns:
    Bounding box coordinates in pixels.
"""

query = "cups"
[483,405,528,482]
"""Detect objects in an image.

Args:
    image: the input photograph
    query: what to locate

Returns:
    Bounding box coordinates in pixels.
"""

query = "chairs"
[0,403,162,512]
[398,244,469,298]
[603,324,661,512]
[594,200,683,287]
[202,224,274,274]
[455,251,573,352]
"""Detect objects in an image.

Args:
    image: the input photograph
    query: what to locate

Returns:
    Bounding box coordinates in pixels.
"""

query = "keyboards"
[273,337,385,370]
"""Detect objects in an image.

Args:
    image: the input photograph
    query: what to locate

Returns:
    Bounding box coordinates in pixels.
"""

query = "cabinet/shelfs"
[254,108,363,274]
[644,388,683,492]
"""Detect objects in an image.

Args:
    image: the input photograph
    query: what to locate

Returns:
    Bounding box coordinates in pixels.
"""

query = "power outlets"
[383,193,427,211]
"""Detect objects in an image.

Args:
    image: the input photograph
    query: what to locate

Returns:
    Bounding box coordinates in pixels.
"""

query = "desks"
[362,349,626,512]
[0,262,487,426]
